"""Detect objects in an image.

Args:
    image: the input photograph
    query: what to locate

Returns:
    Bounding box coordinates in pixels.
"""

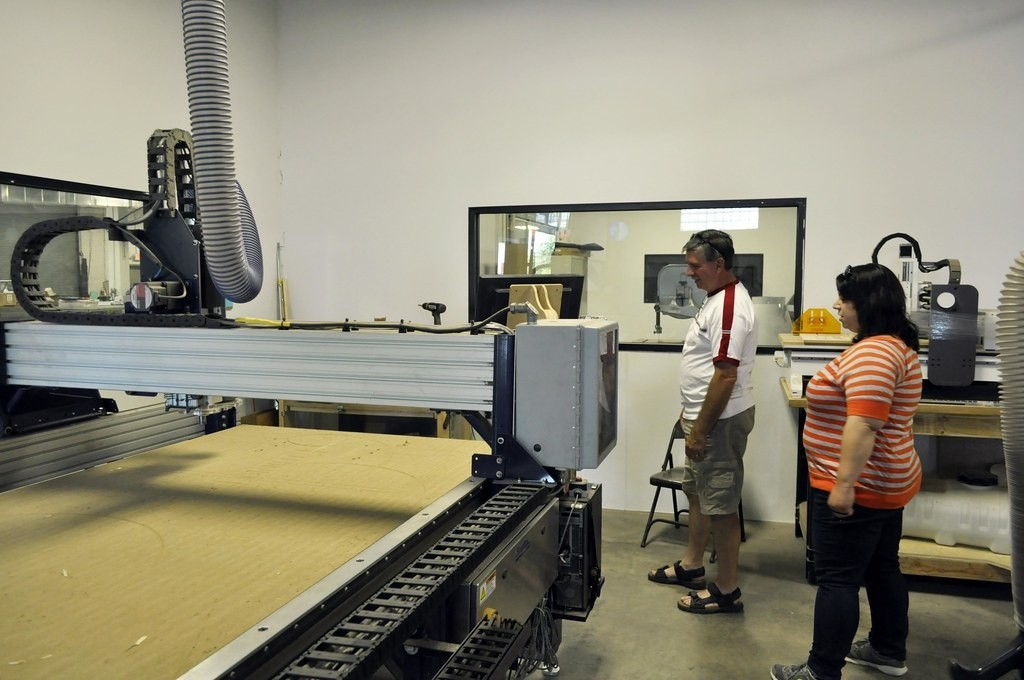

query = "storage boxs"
[241,409,278,426]
[551,254,587,276]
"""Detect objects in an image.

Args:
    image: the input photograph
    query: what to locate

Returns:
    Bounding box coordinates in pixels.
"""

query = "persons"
[648,229,757,613]
[771,264,922,680]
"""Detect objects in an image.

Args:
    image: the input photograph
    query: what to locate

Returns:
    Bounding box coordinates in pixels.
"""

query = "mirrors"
[467,197,807,355]
[0,171,149,321]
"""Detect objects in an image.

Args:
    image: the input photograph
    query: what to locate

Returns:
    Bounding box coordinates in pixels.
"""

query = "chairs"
[640,418,746,564]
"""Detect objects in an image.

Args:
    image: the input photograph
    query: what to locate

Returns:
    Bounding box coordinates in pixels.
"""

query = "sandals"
[648,560,706,590]
[678,582,744,613]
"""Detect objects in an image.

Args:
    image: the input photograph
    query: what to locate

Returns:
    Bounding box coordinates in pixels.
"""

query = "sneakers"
[845,638,908,676]
[770,661,823,680]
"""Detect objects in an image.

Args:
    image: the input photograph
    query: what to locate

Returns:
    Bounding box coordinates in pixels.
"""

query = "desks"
[279,399,475,440]
[778,376,1012,586]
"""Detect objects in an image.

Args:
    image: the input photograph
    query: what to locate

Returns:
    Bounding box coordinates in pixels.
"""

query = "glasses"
[690,234,725,258]
